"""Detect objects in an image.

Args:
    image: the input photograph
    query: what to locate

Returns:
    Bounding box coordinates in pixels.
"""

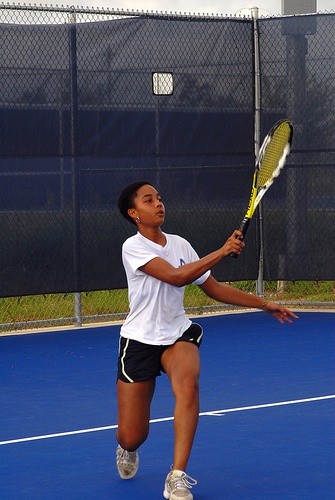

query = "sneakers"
[163,470,198,500]
[117,444,139,479]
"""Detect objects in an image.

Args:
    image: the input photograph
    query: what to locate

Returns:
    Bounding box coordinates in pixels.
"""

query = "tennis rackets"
[229,120,294,259]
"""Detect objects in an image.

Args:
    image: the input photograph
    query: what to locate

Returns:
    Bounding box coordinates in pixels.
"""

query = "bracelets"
[261,298,268,308]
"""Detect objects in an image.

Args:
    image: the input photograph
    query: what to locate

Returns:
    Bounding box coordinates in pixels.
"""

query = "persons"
[114,179,300,500]
